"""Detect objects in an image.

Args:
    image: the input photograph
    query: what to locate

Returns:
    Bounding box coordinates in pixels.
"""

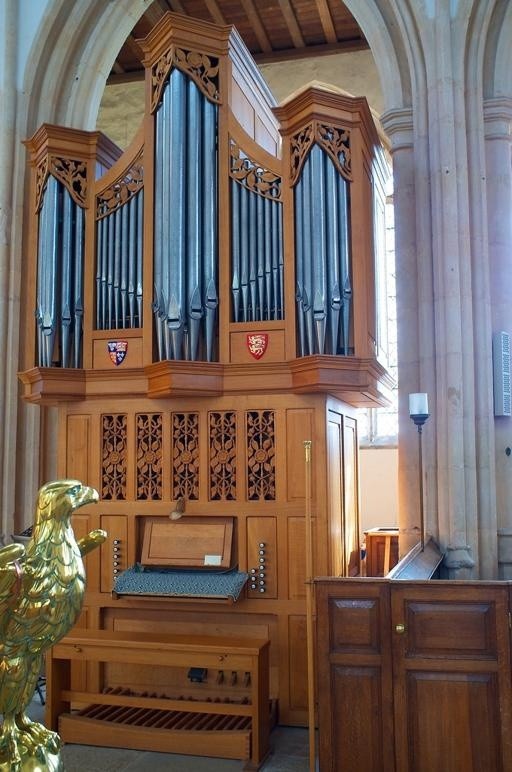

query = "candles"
[408,393,430,415]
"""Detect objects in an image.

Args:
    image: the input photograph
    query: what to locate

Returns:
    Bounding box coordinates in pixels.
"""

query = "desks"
[45,629,271,772]
[363,527,399,577]
